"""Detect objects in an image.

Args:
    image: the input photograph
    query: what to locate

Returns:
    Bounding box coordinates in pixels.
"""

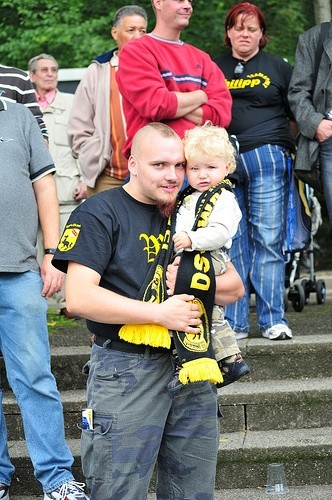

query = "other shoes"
[236,332,249,339]
[216,354,250,389]
[262,323,293,339]
[167,354,190,392]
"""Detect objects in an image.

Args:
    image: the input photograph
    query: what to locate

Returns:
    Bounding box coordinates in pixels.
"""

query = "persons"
[0,0,332,340]
[52,122,244,500]
[0,90,90,500]
[164,118,251,398]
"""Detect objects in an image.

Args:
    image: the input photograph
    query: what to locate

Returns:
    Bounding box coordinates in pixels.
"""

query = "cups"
[265,463,289,496]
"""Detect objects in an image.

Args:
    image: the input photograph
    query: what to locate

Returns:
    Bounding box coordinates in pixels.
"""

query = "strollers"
[248,141,327,313]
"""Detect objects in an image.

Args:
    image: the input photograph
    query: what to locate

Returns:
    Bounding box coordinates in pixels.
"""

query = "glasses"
[233,60,247,80]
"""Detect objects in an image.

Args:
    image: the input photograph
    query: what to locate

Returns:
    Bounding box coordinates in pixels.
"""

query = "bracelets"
[43,248,56,254]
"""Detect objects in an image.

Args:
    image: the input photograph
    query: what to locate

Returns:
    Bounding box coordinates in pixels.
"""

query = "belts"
[94,334,170,354]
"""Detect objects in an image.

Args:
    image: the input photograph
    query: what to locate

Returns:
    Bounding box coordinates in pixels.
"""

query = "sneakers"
[43,479,88,500]
[0,484,10,500]
